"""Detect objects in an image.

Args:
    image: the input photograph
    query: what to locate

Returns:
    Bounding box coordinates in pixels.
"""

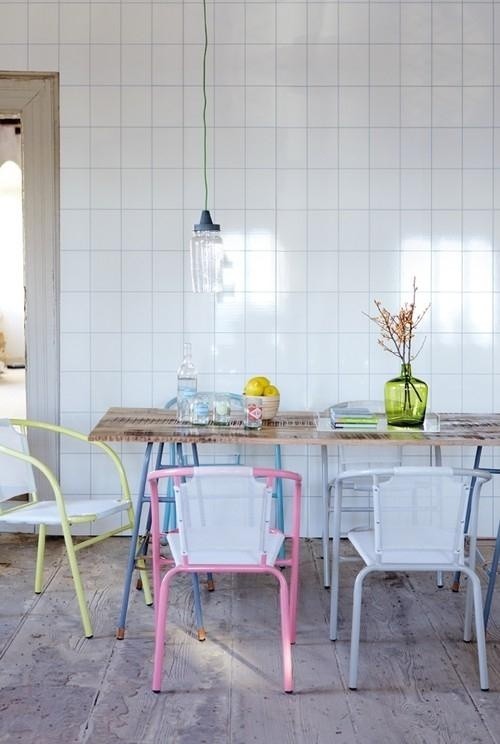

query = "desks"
[87,404,500,641]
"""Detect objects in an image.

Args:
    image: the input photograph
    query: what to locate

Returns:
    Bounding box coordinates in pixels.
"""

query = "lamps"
[187,1,226,298]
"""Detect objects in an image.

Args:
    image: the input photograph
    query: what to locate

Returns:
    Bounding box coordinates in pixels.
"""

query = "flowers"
[361,272,433,412]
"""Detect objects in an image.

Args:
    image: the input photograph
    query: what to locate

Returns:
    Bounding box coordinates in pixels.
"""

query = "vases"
[384,362,427,428]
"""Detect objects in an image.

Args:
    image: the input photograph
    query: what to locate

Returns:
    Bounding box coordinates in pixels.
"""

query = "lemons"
[246,379,264,396]
[263,385,277,396]
[249,376,269,387]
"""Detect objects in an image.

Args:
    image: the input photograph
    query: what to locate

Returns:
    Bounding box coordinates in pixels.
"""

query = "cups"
[212,392,231,424]
[191,393,209,424]
[243,398,264,428]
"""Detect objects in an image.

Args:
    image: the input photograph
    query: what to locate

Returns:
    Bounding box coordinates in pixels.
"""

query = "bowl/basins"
[242,394,280,421]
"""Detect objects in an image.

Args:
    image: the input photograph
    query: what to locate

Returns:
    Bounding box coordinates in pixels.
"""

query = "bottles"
[176,342,197,423]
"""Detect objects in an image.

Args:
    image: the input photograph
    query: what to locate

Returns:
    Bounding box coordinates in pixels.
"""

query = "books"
[330,407,378,430]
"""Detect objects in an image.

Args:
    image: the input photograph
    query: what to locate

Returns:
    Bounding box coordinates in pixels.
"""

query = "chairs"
[0,418,153,638]
[166,393,498,637]
[329,468,489,693]
[147,466,302,694]
